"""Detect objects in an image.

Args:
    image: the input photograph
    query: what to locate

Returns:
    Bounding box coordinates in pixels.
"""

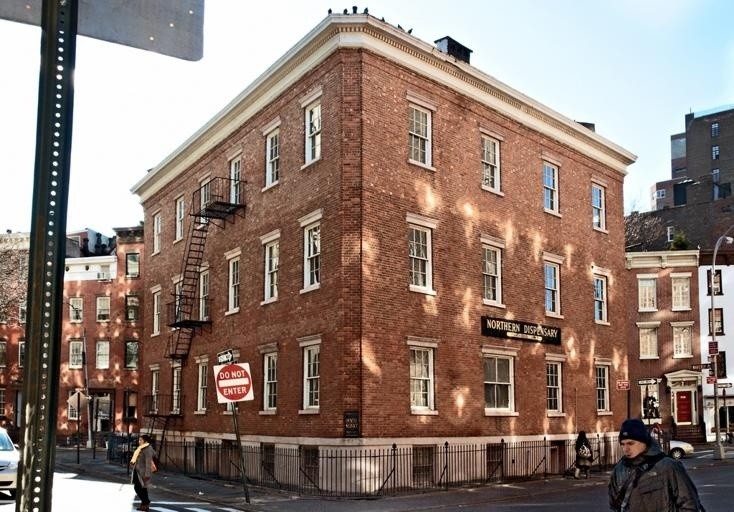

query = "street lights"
[710,236,734,459]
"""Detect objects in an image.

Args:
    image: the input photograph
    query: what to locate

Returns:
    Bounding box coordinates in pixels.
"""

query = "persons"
[128,434,159,512]
[652,422,664,451]
[606,418,703,512]
[574,430,593,480]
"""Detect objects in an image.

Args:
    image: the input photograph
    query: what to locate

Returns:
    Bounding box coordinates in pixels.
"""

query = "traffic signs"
[716,383,732,389]
[637,378,657,385]
[690,364,715,371]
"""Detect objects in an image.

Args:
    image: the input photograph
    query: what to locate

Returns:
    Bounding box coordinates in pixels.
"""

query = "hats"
[618,418,650,442]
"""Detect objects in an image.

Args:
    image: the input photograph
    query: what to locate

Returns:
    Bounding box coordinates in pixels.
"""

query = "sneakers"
[136,502,149,510]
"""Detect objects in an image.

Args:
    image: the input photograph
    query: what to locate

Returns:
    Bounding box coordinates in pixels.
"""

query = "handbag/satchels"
[150,459,157,473]
[578,445,591,458]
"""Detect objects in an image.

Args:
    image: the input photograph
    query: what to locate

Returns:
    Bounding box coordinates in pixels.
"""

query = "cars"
[117,436,138,460]
[0,426,21,496]
[658,438,694,461]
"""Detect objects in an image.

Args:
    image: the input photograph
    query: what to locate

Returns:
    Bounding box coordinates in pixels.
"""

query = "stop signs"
[213,363,255,404]
